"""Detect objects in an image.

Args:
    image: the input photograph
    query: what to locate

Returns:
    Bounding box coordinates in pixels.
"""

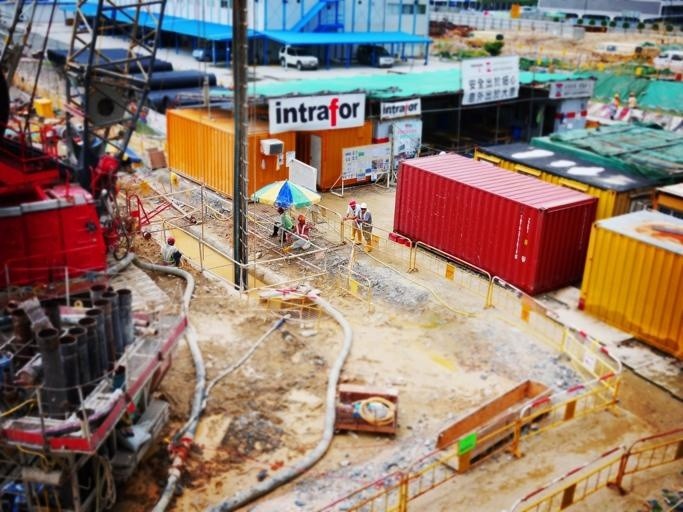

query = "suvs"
[277,45,319,70]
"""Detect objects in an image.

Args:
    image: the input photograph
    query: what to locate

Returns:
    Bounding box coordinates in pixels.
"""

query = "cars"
[192,41,231,61]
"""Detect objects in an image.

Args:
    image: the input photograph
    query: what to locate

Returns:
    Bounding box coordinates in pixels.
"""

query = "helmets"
[277,207,284,213]
[360,202,367,208]
[348,200,356,206]
[298,215,305,223]
[168,237,175,245]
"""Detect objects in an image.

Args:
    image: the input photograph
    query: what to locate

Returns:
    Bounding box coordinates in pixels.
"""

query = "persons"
[283,215,315,253]
[629,93,636,107]
[269,208,294,241]
[162,237,181,266]
[610,94,620,120]
[343,200,362,245]
[356,203,372,252]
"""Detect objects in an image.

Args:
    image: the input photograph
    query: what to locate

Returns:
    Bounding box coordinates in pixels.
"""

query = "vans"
[595,42,641,55]
[651,48,682,73]
[355,44,394,68]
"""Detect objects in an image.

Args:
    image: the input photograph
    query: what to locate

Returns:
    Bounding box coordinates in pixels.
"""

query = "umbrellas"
[251,179,321,215]
